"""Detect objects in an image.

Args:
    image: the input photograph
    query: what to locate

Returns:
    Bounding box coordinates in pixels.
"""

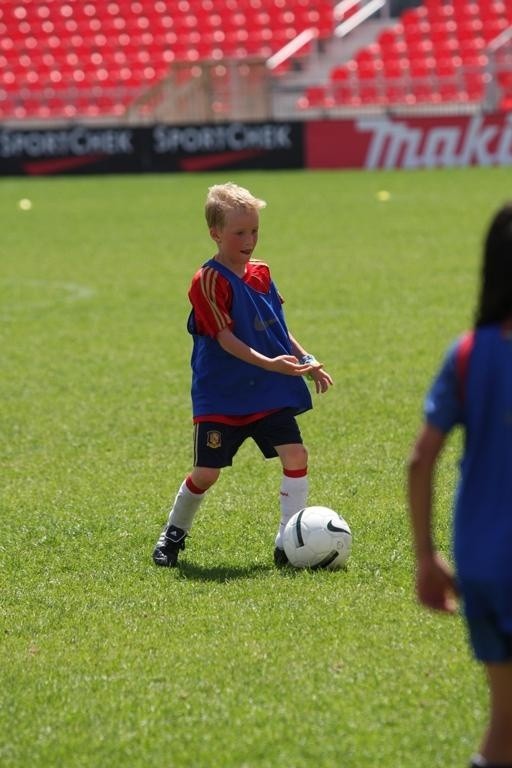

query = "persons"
[152,180,337,569]
[402,200,510,768]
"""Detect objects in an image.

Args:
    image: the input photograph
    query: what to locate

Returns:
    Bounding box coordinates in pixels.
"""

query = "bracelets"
[299,354,323,382]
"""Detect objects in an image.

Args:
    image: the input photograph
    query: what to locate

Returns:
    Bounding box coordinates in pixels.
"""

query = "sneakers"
[153,525,188,567]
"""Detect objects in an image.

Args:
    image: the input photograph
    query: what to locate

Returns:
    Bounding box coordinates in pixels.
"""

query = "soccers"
[282,505,352,571]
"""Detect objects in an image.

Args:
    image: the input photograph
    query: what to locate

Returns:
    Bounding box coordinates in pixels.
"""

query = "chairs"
[0,0,512,125]
